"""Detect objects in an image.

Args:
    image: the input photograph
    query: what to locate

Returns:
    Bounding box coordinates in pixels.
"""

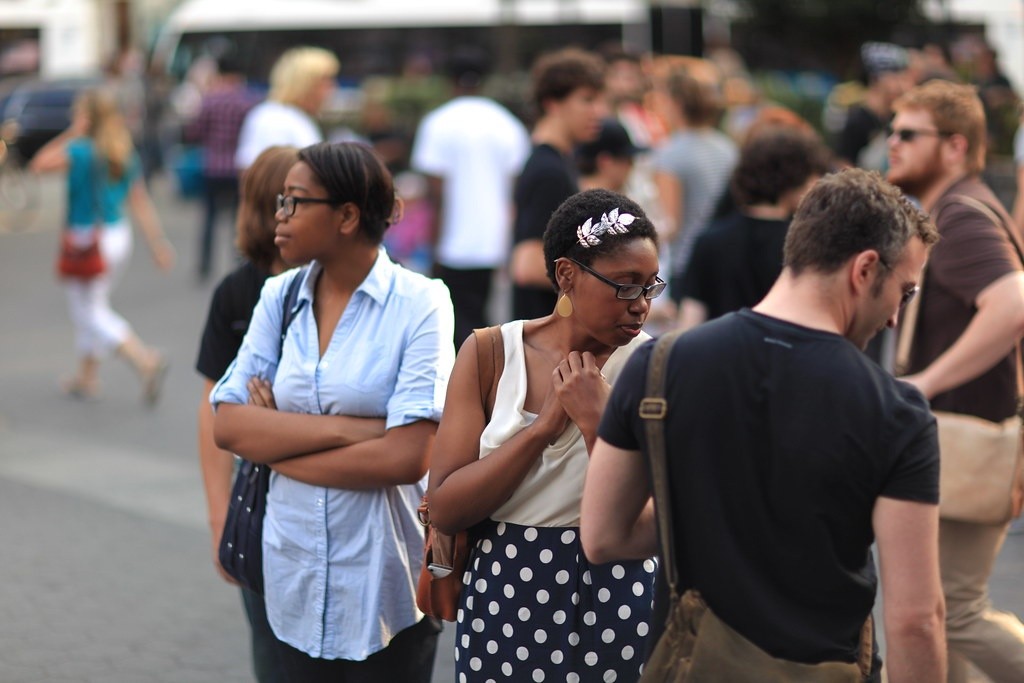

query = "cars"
[1,74,108,167]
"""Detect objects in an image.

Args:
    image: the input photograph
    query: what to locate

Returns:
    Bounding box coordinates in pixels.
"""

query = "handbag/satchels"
[58,239,102,277]
[636,591,866,683]
[927,408,1024,524]
[217,457,274,592]
[416,490,471,623]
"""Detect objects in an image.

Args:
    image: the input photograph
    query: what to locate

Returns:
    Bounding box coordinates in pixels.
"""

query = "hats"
[578,116,651,156]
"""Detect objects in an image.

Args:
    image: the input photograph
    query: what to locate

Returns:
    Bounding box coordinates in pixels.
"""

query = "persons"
[25,29,1023,406]
[883,81,1024,683]
[206,138,457,682]
[191,146,293,682]
[428,190,662,683]
[582,168,951,682]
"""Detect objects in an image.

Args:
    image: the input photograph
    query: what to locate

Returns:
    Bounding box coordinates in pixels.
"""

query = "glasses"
[885,124,952,143]
[553,258,666,300]
[276,193,330,217]
[876,255,919,311]
